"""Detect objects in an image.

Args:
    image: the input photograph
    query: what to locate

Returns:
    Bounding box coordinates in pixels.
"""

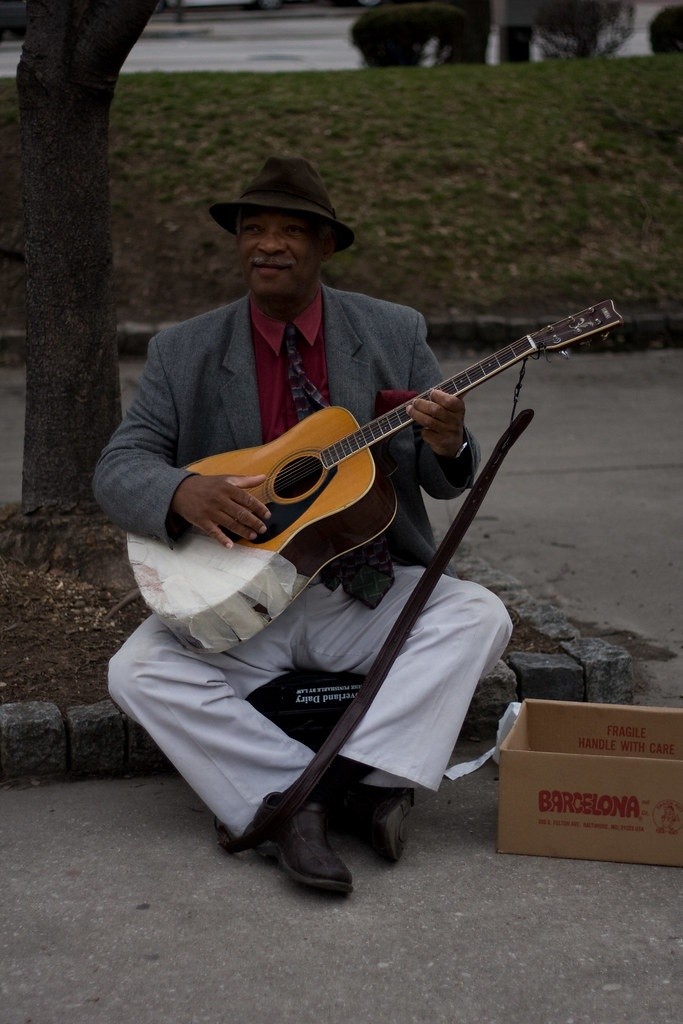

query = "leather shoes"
[261,790,354,892]
[353,785,416,863]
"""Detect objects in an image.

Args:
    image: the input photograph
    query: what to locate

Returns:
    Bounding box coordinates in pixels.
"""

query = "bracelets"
[454,441,468,458]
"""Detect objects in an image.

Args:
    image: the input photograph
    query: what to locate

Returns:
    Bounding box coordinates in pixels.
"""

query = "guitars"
[126,299,626,653]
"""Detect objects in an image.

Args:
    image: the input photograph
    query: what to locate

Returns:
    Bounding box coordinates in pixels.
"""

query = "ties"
[280,322,394,610]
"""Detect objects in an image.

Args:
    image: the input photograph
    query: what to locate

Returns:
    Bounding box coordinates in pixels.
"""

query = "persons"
[494,0,541,63]
[91,157,514,898]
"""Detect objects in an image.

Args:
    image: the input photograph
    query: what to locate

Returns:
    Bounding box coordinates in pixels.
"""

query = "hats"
[207,155,356,251]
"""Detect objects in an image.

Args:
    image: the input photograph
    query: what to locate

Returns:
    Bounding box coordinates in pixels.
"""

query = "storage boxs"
[496,698,683,867]
